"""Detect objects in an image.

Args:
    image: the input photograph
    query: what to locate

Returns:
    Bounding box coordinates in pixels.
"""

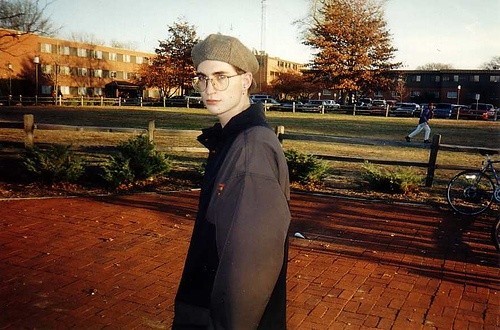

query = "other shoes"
[405,136,410,142]
[424,140,432,144]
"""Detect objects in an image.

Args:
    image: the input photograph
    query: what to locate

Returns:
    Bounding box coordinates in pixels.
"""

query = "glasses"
[191,73,243,92]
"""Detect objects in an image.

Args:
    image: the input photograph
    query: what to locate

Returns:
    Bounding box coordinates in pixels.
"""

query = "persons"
[171,33,291,330]
[405,103,434,143]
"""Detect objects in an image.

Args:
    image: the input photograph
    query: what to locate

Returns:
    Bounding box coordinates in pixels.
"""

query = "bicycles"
[446,150,500,217]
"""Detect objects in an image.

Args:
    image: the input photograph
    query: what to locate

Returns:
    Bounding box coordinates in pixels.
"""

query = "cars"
[141,90,500,121]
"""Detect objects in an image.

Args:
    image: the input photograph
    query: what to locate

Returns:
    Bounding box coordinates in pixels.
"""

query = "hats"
[191,35,260,75]
[429,103,436,106]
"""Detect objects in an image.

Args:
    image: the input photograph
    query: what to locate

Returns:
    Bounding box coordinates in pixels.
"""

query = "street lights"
[8,63,13,106]
[33,56,39,107]
